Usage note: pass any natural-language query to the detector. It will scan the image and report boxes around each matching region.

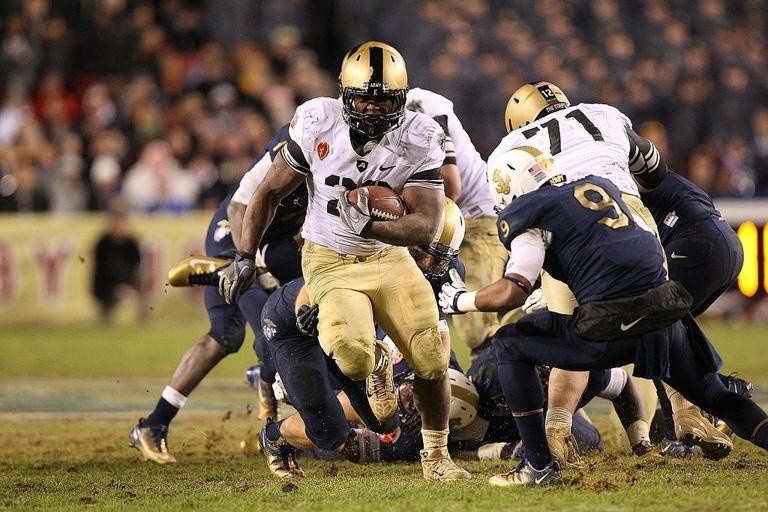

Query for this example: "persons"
[127,40,768,488]
[88,211,143,323]
[0,0,767,209]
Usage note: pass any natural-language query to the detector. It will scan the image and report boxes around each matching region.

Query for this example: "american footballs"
[349,187,404,222]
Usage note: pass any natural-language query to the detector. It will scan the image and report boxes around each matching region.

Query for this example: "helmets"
[341,41,408,139]
[395,368,478,433]
[487,146,566,216]
[408,196,465,280]
[504,81,571,133]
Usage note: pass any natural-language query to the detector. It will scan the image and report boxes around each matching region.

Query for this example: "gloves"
[296,304,319,337]
[438,268,468,315]
[522,287,547,315]
[218,251,256,305]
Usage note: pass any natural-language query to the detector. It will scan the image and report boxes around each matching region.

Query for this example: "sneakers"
[478,370,752,489]
[420,447,472,481]
[247,339,399,484]
[128,417,177,465]
[168,255,235,287]
